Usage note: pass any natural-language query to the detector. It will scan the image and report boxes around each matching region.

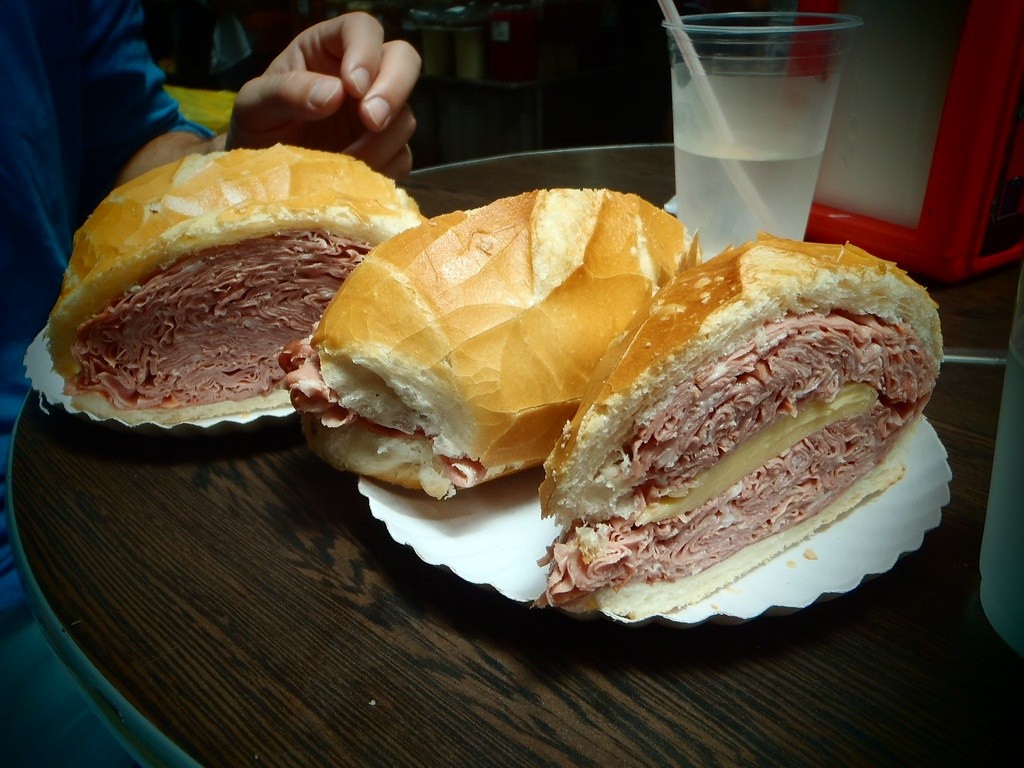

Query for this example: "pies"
[537,231,945,616]
[41,143,429,424]
[276,187,704,495]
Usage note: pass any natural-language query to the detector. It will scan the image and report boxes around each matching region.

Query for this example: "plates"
[22,320,296,430]
[358,414,953,623]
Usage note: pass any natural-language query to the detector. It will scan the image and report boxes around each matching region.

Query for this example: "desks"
[3,141,1024,768]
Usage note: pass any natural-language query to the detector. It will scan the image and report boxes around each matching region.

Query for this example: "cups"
[661,13,865,263]
[978,275,1024,655]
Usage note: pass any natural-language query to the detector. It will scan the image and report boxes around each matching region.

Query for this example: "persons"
[0,0,423,768]
